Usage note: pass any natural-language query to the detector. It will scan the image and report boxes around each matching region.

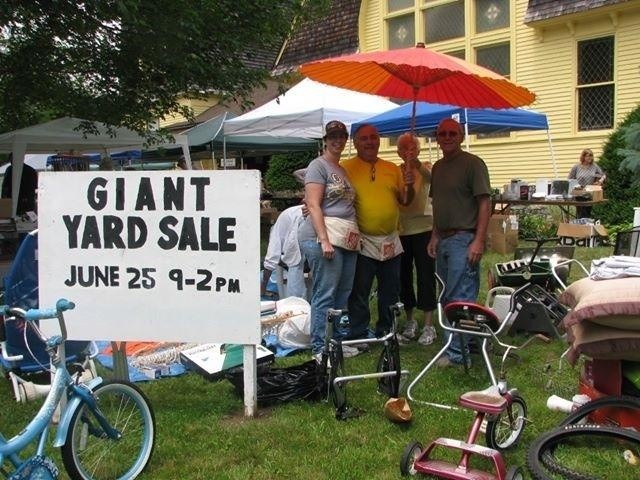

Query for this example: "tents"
[223,78,399,170]
[349,102,558,180]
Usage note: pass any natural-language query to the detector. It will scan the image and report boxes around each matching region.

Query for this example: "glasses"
[438,129,459,138]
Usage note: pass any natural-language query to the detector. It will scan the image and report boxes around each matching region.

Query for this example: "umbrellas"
[300,42,537,204]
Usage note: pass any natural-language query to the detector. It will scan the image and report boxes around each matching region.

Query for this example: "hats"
[324,121,348,136]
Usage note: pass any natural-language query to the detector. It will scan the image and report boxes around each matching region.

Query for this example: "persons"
[568,149,607,219]
[301,116,491,360]
[1,152,38,217]
[260,204,307,301]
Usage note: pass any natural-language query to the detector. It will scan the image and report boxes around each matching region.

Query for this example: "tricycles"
[399,324,551,479]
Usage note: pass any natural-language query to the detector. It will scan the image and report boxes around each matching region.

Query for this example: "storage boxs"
[487,214,518,255]
[556,221,608,248]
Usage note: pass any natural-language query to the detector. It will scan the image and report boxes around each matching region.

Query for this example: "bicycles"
[1,299,157,479]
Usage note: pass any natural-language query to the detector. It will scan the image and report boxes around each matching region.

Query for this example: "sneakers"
[311,320,472,369]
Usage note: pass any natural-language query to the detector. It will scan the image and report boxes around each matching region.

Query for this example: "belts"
[437,228,475,239]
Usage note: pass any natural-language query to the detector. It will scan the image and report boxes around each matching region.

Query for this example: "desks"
[492,196,609,222]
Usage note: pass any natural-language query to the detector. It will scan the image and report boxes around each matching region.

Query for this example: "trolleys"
[486,232,566,299]
[319,299,406,417]
